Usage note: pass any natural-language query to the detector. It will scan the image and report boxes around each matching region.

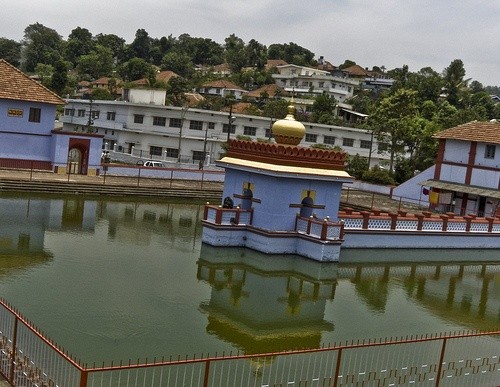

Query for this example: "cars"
[144,160,164,167]
